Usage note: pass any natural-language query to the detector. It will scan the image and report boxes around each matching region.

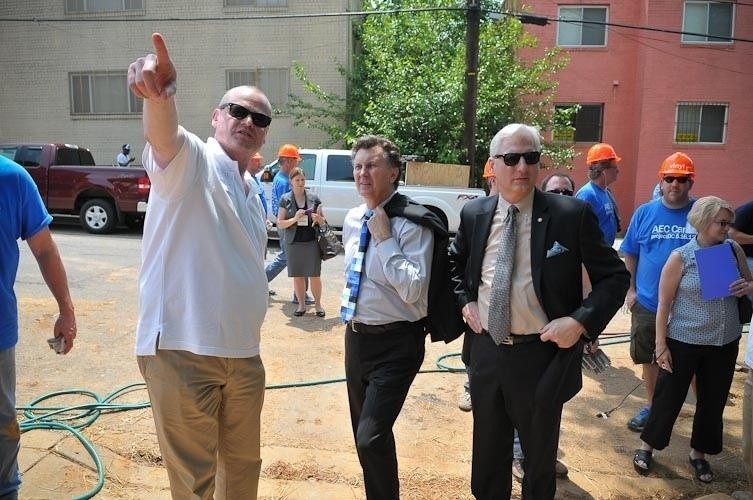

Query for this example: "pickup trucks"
[0,144,152,229]
[256,150,486,234]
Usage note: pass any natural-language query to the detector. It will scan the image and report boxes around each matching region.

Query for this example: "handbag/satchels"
[317,230,343,261]
[737,294,753,324]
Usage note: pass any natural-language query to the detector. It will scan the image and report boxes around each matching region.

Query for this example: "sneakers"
[554,459,568,479]
[511,458,525,483]
[458,388,472,411]
[292,293,315,305]
[627,406,651,431]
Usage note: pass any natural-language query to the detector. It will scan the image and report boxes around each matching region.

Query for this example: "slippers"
[632,448,652,476]
[688,454,713,482]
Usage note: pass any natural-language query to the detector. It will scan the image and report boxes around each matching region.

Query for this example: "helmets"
[250,152,263,160]
[657,152,697,176]
[586,143,622,166]
[122,143,130,150]
[482,157,496,179]
[277,144,301,161]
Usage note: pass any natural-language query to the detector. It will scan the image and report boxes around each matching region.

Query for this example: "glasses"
[217,102,271,128]
[491,147,542,166]
[546,188,574,197]
[661,175,691,183]
[714,220,732,227]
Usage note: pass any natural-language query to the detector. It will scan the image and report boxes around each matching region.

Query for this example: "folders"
[694,243,741,300]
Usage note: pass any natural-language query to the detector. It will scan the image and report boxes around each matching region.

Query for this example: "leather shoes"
[315,307,325,316]
[293,306,306,316]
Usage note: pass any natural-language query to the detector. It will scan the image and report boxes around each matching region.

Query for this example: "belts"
[345,320,410,336]
[501,334,540,346]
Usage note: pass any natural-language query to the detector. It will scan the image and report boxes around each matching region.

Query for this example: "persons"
[449,122,632,500]
[632,195,753,482]
[729,204,753,500]
[342,138,434,499]
[573,143,622,356]
[246,144,325,318]
[513,173,574,482]
[620,151,698,429]
[117,144,135,168]
[0,154,78,498]
[459,156,494,412]
[126,32,273,500]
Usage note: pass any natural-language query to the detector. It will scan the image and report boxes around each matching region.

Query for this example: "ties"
[339,208,373,325]
[487,204,520,347]
